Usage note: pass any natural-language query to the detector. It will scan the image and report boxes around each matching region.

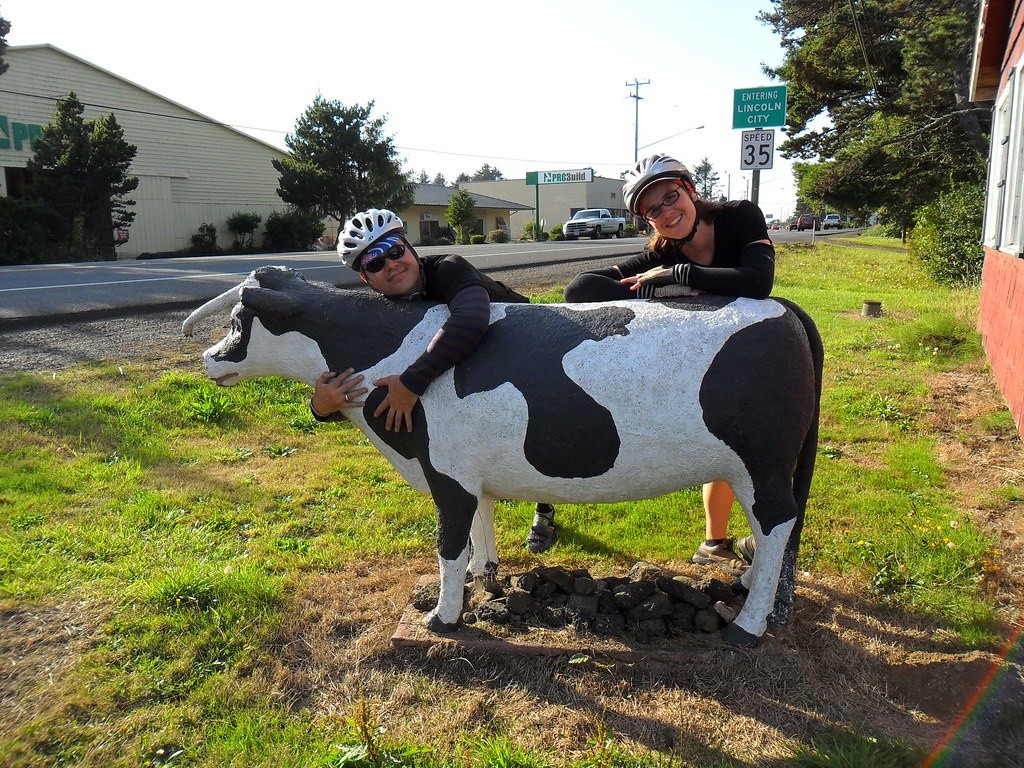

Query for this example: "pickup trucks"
[563,208,626,240]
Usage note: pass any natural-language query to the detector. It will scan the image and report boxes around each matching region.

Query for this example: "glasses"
[644,185,682,223]
[363,243,406,273]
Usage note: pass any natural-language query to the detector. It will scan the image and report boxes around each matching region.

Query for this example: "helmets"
[621,154,695,217]
[337,209,406,273]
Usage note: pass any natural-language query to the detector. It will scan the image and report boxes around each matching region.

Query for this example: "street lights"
[634,125,705,230]
[724,170,730,202]
[743,176,749,201]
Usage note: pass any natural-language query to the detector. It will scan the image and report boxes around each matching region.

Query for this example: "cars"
[771,225,779,230]
[823,214,842,229]
[785,213,821,232]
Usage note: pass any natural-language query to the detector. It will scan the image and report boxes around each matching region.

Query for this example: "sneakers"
[737,536,755,564]
[529,505,555,553]
[692,537,751,576]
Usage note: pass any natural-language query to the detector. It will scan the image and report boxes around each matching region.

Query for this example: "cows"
[180,265,824,647]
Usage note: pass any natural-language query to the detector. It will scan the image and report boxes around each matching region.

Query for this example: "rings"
[345,393,350,402]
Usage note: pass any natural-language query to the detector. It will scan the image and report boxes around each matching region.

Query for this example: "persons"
[305,208,556,553]
[564,152,777,576]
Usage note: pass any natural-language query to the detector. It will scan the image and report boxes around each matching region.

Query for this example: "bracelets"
[310,398,329,417]
